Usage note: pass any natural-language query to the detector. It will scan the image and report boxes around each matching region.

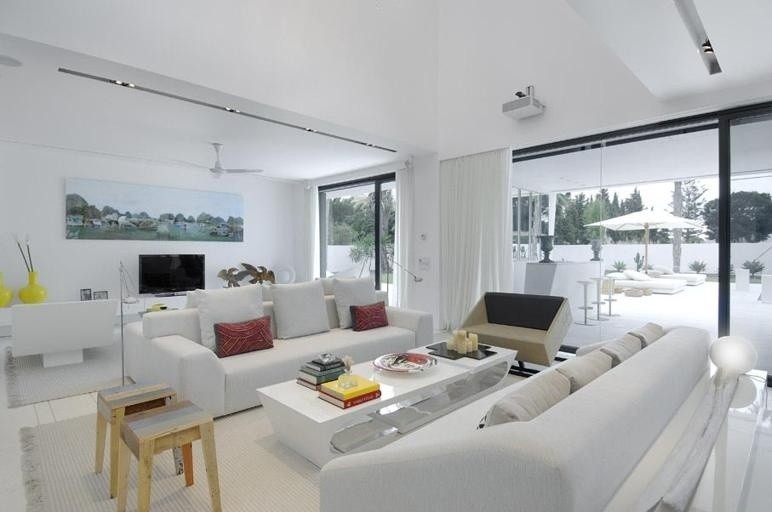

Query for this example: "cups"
[446,329,479,354]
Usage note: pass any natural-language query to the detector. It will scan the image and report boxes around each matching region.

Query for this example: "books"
[296,353,345,391]
[321,374,380,401]
[318,389,381,409]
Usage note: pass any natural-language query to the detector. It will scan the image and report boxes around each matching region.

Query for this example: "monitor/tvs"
[140,253,205,298]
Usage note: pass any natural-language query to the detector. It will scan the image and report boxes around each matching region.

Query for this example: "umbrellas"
[583,204,703,275]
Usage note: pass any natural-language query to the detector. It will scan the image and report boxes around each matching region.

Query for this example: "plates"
[373,353,438,373]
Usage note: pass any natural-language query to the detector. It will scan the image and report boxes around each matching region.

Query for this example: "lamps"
[709,334,762,397]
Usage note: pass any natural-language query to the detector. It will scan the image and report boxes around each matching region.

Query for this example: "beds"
[605,267,710,297]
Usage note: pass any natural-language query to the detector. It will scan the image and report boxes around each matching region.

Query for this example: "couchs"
[315,315,719,512]
[121,275,434,424]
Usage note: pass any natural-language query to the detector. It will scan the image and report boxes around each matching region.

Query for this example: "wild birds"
[241,263,275,284]
[217,268,252,288]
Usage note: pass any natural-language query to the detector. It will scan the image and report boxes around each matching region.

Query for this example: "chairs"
[453,287,573,378]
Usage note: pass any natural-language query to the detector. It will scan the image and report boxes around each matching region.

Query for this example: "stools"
[95,378,222,512]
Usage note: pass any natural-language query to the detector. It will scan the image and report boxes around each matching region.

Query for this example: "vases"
[0,271,49,309]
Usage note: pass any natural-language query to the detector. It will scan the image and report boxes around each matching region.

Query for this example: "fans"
[167,141,265,183]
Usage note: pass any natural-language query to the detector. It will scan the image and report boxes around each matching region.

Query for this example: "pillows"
[213,314,275,360]
[349,300,388,334]
